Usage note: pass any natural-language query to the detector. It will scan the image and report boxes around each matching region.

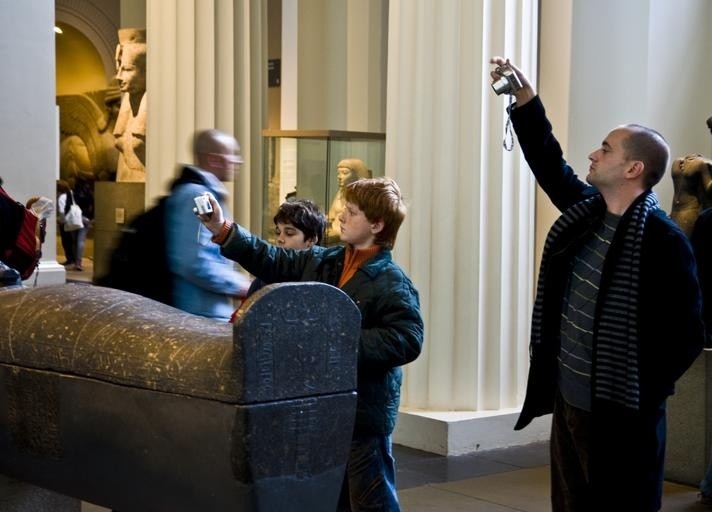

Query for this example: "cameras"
[193,196,213,217]
[491,65,523,95]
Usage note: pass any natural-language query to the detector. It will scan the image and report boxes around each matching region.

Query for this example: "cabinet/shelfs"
[261,128,386,248]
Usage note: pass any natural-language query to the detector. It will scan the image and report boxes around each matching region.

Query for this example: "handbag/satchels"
[64,204,85,232]
[0,187,53,281]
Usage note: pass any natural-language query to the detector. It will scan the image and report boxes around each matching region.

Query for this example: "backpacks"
[97,178,202,304]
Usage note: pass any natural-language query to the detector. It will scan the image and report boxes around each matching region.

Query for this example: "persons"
[487,55,706,510]
[56,180,76,266]
[113,42,146,183]
[166,128,252,320]
[677,151,711,353]
[64,174,96,271]
[321,158,368,239]
[236,195,325,317]
[184,175,425,512]
